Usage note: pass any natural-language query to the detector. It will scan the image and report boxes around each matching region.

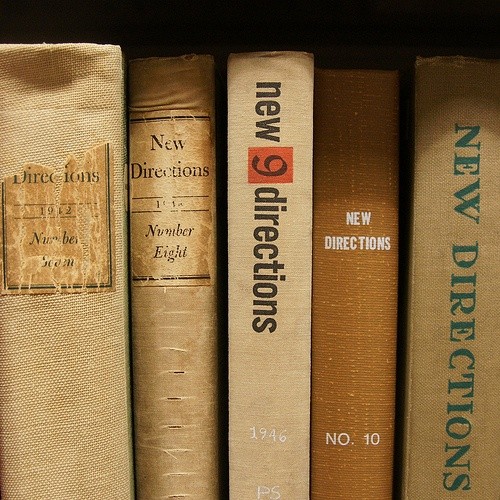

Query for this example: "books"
[0,44,500,498]
